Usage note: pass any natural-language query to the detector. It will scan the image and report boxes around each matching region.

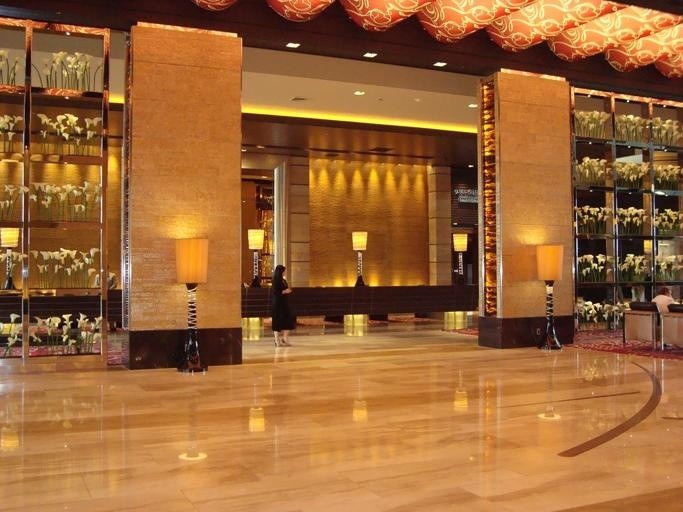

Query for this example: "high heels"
[274,338,291,347]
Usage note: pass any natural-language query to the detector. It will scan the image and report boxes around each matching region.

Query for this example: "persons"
[650,287,677,349]
[93,264,118,289]
[268,346,294,429]
[271,265,296,347]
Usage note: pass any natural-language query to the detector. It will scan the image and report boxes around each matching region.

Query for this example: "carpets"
[0,340,128,366]
[448,327,682,360]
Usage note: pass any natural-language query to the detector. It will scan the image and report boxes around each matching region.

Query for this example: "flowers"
[0,17,112,368]
[572,87,683,333]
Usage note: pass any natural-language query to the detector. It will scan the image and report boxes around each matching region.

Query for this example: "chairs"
[621,300,683,354]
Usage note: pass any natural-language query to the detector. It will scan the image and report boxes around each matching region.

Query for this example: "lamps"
[351,231,368,287]
[174,237,209,372]
[453,233,467,285]
[536,245,564,350]
[246,228,264,287]
